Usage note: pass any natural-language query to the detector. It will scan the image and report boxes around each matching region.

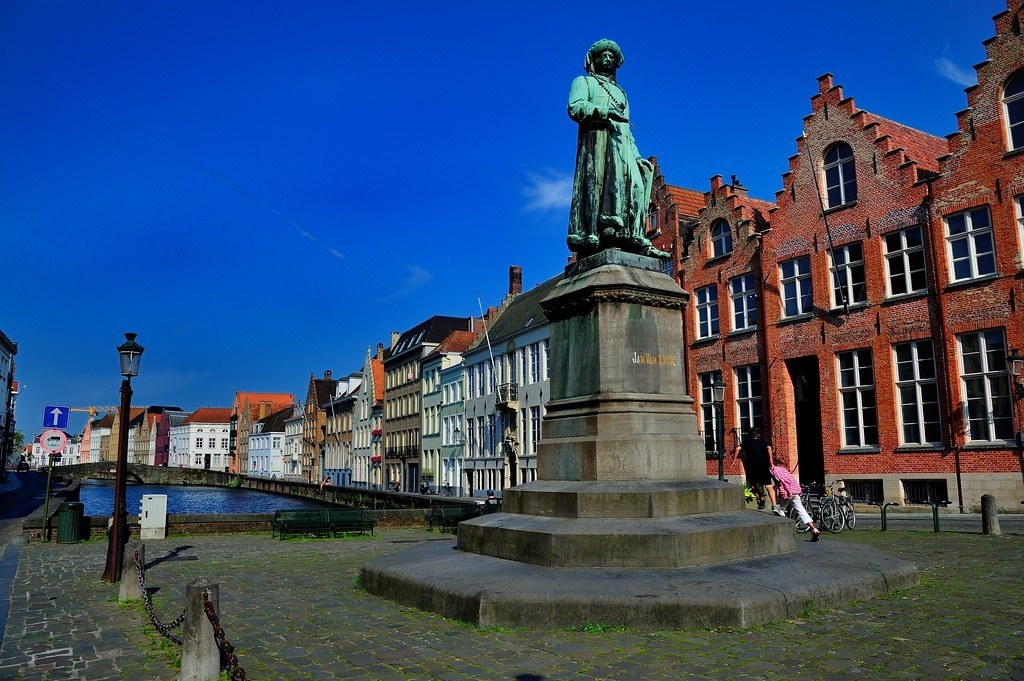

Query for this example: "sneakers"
[756,499,765,510]
[771,505,786,516]
[811,528,820,542]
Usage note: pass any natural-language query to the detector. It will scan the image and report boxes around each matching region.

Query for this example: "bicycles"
[818,479,857,534]
[789,481,822,533]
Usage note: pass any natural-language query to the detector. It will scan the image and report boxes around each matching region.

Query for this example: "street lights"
[102,332,144,579]
[710,375,727,481]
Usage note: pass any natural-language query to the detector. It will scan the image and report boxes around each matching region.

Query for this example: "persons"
[394,481,401,492]
[320,475,331,491]
[476,489,502,509]
[769,463,821,543]
[567,38,672,259]
[729,424,786,516]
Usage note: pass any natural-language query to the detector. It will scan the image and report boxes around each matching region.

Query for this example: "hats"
[486,489,494,495]
[327,477,331,479]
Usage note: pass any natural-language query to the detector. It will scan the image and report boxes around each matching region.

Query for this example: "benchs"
[424,501,502,535]
[271,508,378,541]
